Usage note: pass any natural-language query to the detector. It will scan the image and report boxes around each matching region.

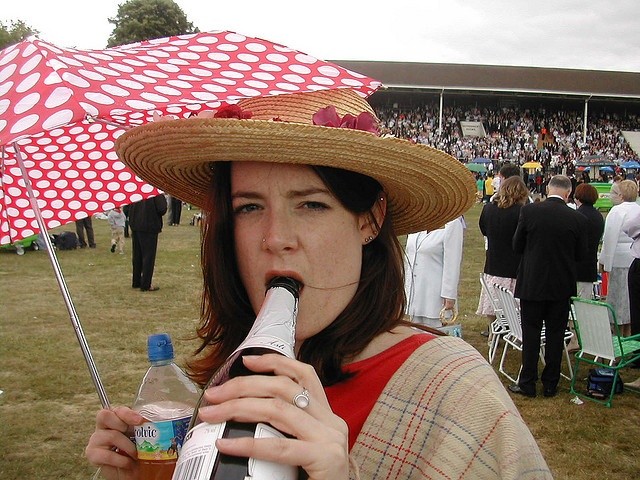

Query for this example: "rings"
[292,387,310,409]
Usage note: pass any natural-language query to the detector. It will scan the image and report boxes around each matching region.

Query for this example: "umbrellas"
[473,157,493,164]
[1,30,385,413]
[618,160,640,169]
[465,162,488,174]
[598,165,614,173]
[521,159,545,171]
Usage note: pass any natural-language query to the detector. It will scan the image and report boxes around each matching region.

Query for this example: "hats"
[115,88,476,236]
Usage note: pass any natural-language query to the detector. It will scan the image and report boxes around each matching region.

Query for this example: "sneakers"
[111,243,116,253]
[150,286,159,290]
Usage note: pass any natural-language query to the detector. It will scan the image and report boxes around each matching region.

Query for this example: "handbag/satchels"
[435,323,461,338]
[587,368,623,398]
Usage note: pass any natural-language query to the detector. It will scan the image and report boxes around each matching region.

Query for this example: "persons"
[620,211,640,392]
[119,201,131,239]
[566,183,605,354]
[600,179,640,366]
[402,215,467,334]
[170,192,182,226]
[83,89,554,479]
[166,192,173,227]
[387,105,437,147]
[506,175,589,398]
[567,104,640,160]
[122,193,168,292]
[475,175,530,347]
[476,175,485,202]
[484,172,497,203]
[107,206,126,254]
[437,102,566,158]
[76,214,97,250]
[610,175,625,207]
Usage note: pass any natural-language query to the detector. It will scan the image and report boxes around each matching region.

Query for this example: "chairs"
[493,283,575,385]
[478,275,546,365]
[568,295,640,408]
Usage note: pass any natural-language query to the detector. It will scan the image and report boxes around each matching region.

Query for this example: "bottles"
[131,333,203,480]
[171,277,299,480]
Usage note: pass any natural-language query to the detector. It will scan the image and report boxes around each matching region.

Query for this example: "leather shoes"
[508,384,536,395]
